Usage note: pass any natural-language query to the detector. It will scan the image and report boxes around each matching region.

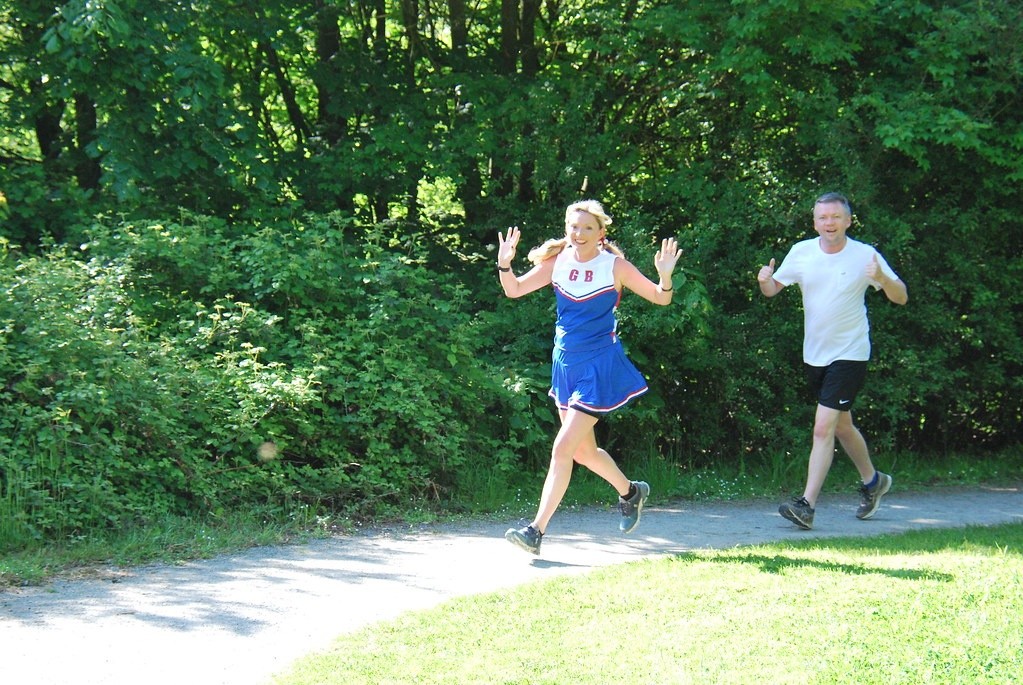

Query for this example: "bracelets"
[661,287,672,291]
[498,266,510,272]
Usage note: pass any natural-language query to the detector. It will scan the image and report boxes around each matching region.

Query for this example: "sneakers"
[612,481,650,533]
[779,496,815,530]
[855,471,891,518]
[506,525,544,555]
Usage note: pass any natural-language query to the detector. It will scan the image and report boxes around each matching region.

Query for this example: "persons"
[498,200,683,556]
[758,192,908,531]
